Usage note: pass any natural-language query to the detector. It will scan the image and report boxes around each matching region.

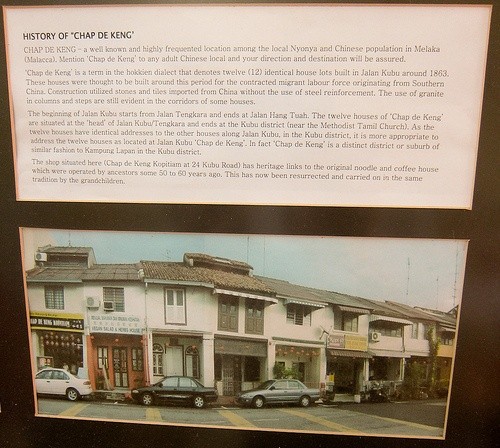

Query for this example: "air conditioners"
[103,301,115,310]
[34,252,48,262]
[369,332,381,342]
[85,295,100,309]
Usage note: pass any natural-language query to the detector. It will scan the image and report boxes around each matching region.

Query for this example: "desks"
[367,389,384,402]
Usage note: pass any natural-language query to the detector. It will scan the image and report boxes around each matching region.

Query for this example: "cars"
[131,376,220,408]
[36,366,92,401]
[236,378,321,410]
